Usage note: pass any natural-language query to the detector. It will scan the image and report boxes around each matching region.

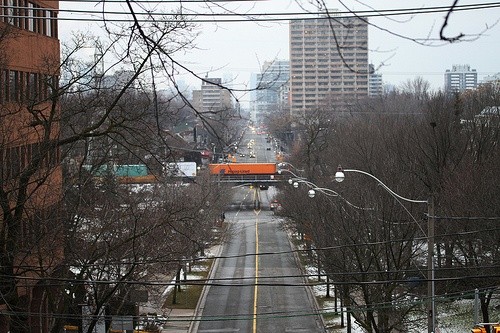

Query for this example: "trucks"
[162,162,206,179]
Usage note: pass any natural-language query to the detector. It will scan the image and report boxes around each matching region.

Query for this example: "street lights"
[308,186,384,270]
[333,164,436,333]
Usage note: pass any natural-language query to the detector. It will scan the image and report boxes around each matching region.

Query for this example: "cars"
[237,118,273,160]
[260,186,269,190]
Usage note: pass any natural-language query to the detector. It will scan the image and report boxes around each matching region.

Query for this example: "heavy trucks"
[209,162,294,181]
[80,163,158,179]
